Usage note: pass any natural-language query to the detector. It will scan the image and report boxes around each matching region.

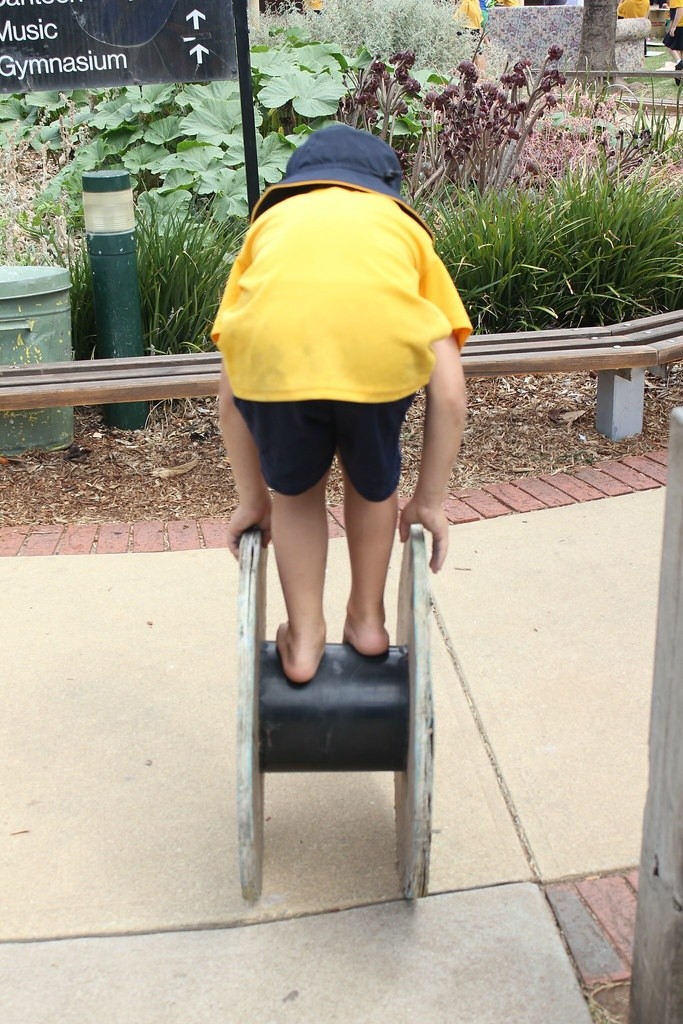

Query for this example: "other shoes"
[675,78,680,85]
[676,60,683,70]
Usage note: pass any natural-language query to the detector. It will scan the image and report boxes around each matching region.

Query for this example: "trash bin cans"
[0,266,75,458]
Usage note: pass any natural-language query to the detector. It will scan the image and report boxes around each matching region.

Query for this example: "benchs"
[514,70,683,115]
[0,309,683,441]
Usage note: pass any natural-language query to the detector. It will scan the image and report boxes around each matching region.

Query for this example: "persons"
[449,0,522,72]
[303,0,323,16]
[618,0,650,19]
[664,0,683,85]
[210,120,469,685]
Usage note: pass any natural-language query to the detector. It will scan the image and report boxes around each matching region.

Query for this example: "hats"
[251,125,434,243]
